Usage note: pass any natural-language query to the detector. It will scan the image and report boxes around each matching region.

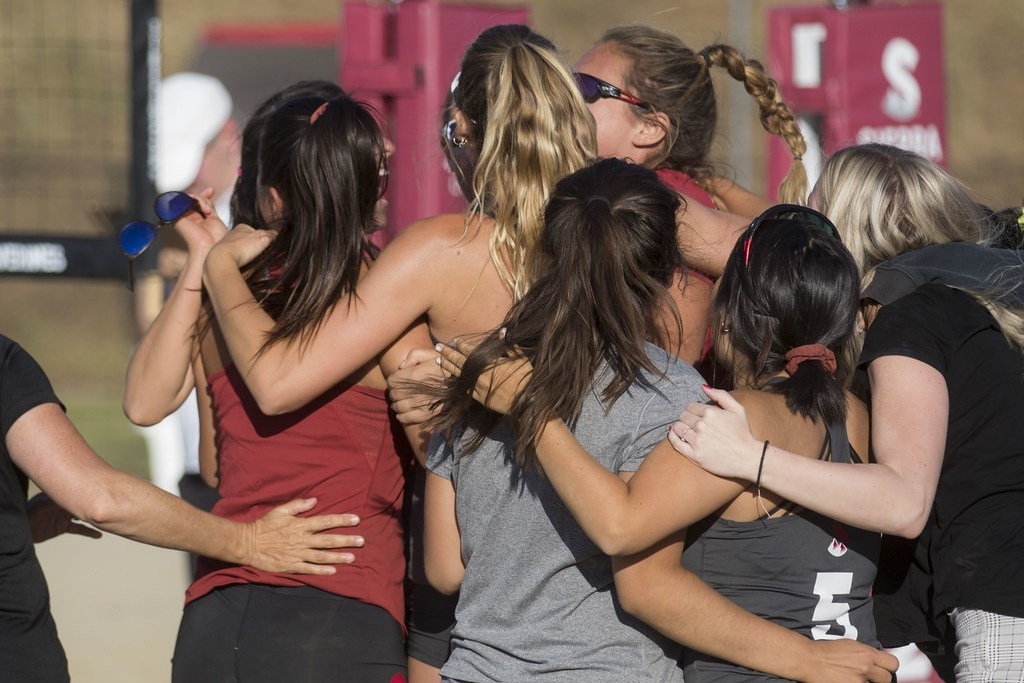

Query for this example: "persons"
[125,21,1024,683]
[0,333,365,683]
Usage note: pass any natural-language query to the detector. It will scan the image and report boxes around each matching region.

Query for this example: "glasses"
[118,191,205,290]
[744,203,843,266]
[574,73,650,110]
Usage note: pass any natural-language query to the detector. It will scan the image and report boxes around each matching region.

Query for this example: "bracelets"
[756,441,770,487]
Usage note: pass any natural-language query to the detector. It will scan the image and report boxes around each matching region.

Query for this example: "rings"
[682,429,692,442]
